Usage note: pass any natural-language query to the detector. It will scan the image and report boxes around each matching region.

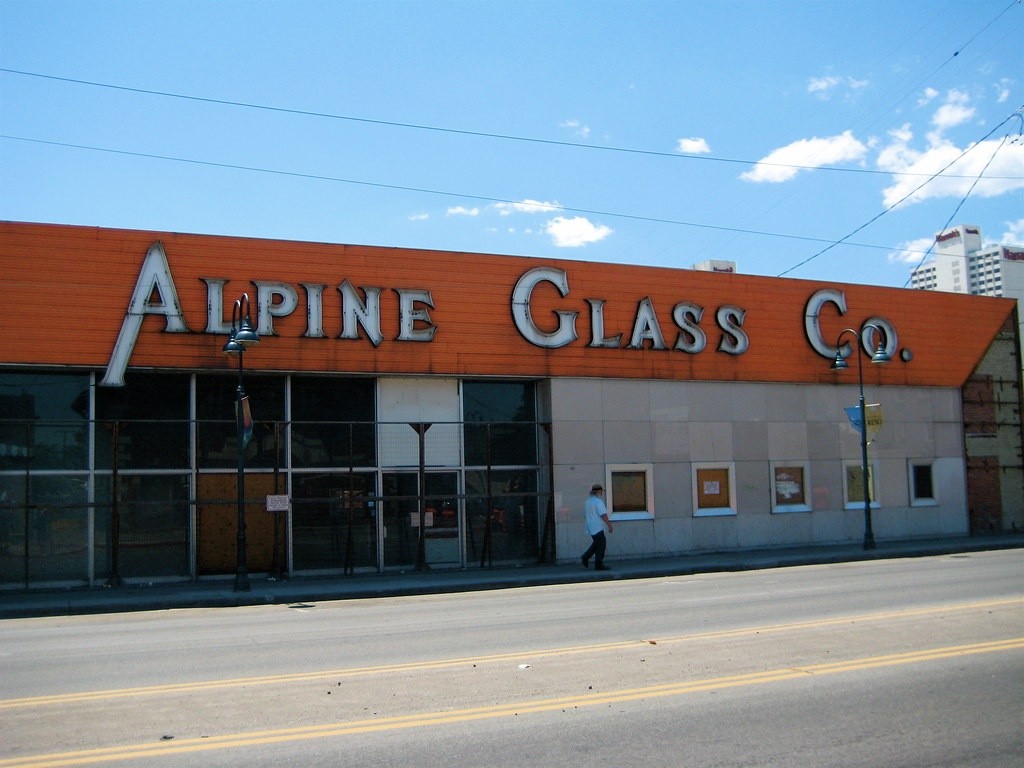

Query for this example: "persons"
[581,484,613,569]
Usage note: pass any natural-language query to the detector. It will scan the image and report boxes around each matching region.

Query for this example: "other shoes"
[595,565,610,570]
[581,555,588,568]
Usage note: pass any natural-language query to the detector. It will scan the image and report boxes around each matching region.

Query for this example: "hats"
[589,485,605,493]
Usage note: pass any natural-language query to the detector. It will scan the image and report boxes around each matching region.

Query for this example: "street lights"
[222,293,262,592]
[830,324,892,550]
